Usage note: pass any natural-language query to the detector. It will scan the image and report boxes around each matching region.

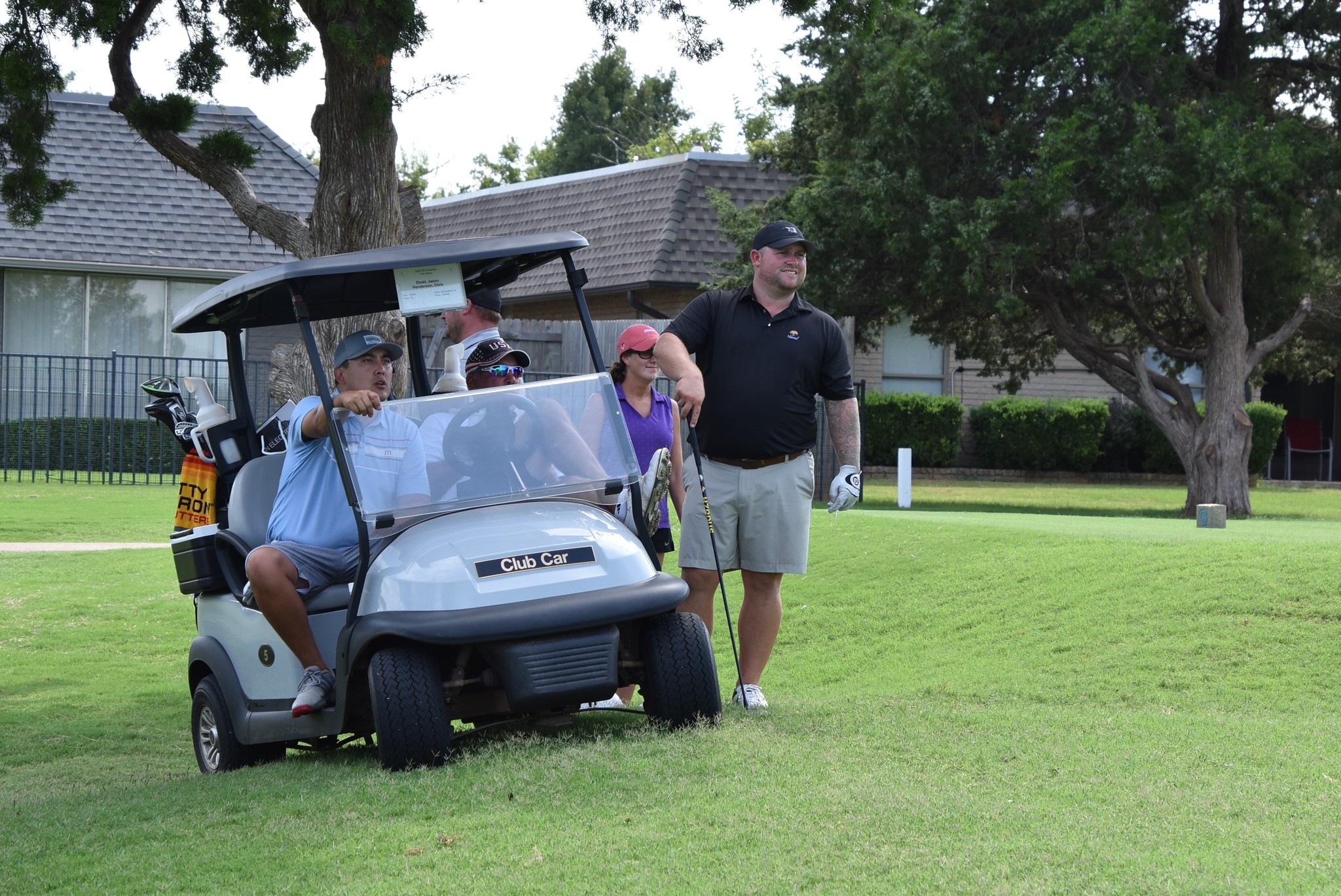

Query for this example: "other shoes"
[579,693,626,712]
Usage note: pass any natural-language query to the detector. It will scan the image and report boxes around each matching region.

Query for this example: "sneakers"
[624,447,673,536]
[732,684,768,709]
[292,665,338,718]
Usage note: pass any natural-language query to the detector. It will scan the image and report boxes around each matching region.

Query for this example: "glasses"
[627,347,654,359]
[469,364,525,378]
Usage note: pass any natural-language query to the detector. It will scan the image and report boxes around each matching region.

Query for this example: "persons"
[434,280,525,411]
[639,220,862,710]
[397,339,672,539]
[245,330,431,718]
[579,324,685,712]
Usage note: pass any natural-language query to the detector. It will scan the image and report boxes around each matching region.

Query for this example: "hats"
[753,220,816,254]
[333,330,404,369]
[464,282,501,313]
[464,339,531,376]
[617,324,660,357]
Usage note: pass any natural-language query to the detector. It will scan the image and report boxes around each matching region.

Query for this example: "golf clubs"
[173,421,221,478]
[686,430,773,717]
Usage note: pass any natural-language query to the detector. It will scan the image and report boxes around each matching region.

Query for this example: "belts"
[697,450,807,470]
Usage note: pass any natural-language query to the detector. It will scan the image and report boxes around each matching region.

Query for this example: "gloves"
[827,465,862,514]
